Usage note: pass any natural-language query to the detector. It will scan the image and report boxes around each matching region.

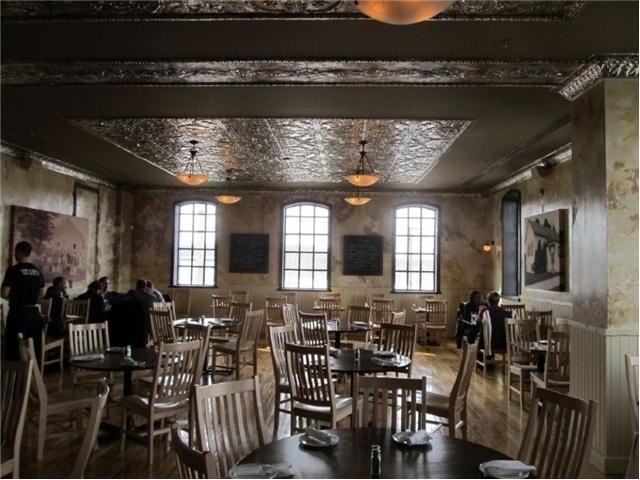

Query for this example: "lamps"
[216,169,241,204]
[483,240,494,252]
[342,140,378,187]
[176,140,207,185]
[345,187,371,205]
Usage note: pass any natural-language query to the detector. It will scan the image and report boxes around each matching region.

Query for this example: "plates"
[479,462,529,479]
[298,434,339,447]
[392,431,433,446]
[229,463,278,479]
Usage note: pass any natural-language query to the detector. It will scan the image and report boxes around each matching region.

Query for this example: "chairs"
[405,349,476,439]
[318,297,343,321]
[378,323,417,398]
[418,296,434,323]
[224,303,252,337]
[270,325,301,437]
[623,355,639,477]
[527,310,554,330]
[181,325,210,371]
[230,290,248,304]
[390,310,407,323]
[281,293,297,304]
[68,321,109,353]
[195,376,267,479]
[120,338,205,468]
[348,305,372,346]
[149,310,176,345]
[476,309,506,376]
[284,345,353,435]
[18,334,108,459]
[35,298,65,377]
[282,304,298,325]
[422,300,449,348]
[517,386,599,478]
[354,375,428,432]
[153,302,179,338]
[506,318,540,408]
[267,297,287,344]
[213,310,264,379]
[299,311,327,356]
[63,298,92,323]
[212,295,235,317]
[72,380,110,478]
[503,303,525,318]
[370,298,394,325]
[0,360,33,479]
[171,426,214,479]
[173,289,193,318]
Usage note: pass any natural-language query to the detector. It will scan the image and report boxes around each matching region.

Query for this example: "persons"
[468,293,506,371]
[456,291,489,349]
[1,241,46,373]
[124,278,158,319]
[99,277,111,304]
[146,281,165,303]
[76,281,106,324]
[41,275,69,337]
